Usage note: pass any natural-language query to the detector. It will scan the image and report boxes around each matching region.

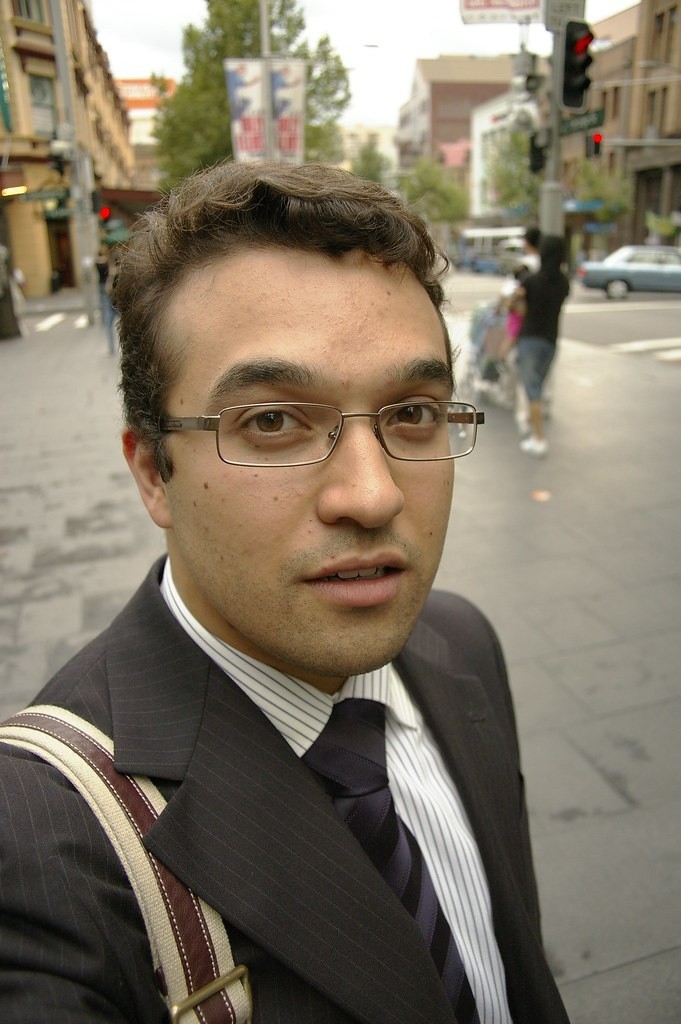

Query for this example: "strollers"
[470,291,521,410]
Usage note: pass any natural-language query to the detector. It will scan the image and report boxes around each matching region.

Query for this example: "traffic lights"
[592,130,602,154]
[98,207,112,231]
[554,17,594,115]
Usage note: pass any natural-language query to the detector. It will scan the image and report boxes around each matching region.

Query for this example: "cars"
[497,238,570,278]
[578,245,681,300]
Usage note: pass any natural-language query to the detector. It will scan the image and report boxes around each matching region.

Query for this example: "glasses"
[140,400,485,469]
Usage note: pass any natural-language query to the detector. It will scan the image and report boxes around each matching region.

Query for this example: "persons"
[88,227,571,453]
[2,162,573,1023]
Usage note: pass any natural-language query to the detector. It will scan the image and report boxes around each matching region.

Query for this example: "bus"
[450,226,532,276]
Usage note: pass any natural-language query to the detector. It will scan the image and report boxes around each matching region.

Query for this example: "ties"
[302,695,482,1023]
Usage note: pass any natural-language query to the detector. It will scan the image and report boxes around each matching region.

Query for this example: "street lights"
[258,1,380,167]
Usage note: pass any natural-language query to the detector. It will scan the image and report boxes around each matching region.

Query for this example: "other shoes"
[521,437,548,457]
